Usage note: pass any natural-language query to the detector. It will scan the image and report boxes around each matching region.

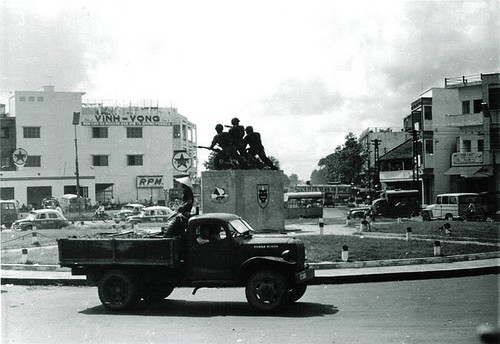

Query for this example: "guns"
[193,146,220,153]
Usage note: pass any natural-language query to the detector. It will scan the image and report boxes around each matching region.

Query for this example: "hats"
[176,177,193,187]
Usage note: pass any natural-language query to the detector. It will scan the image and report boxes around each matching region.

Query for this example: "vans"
[59,194,84,213]
[371,189,421,219]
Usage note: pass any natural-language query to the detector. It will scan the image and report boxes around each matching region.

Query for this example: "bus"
[283,191,325,219]
[286,182,353,203]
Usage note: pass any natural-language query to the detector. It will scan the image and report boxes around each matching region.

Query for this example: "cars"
[124,206,175,226]
[10,208,71,232]
[421,192,478,222]
[0,200,21,229]
[112,204,145,223]
[43,199,57,211]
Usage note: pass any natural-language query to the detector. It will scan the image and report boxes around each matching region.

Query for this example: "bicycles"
[90,211,109,223]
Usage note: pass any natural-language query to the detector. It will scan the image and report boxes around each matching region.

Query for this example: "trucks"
[56,212,315,312]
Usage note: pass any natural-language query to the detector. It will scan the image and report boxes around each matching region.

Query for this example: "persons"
[228,118,264,170]
[175,177,194,215]
[110,196,115,209]
[208,124,252,170]
[244,126,279,169]
[56,203,63,214]
[95,203,104,216]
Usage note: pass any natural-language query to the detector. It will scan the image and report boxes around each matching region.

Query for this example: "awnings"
[443,167,492,177]
